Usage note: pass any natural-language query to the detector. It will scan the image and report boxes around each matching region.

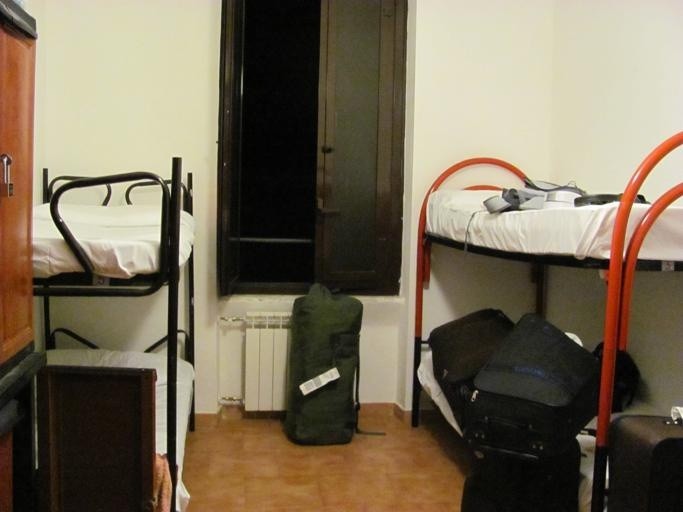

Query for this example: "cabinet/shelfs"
[0,2,38,512]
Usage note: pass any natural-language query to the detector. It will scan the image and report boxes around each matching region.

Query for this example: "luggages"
[609,413,682,512]
[427,306,600,512]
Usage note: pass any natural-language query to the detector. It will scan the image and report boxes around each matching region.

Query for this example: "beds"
[412,133,683,512]
[31,158,195,511]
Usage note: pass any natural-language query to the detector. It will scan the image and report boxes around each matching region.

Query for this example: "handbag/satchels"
[590,339,641,413]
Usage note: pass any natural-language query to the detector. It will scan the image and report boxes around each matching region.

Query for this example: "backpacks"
[283,282,363,449]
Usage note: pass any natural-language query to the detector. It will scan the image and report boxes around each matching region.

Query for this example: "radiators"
[244,313,293,413]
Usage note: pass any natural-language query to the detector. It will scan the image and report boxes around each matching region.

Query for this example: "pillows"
[433,190,503,210]
[43,348,181,385]
[32,200,163,227]
